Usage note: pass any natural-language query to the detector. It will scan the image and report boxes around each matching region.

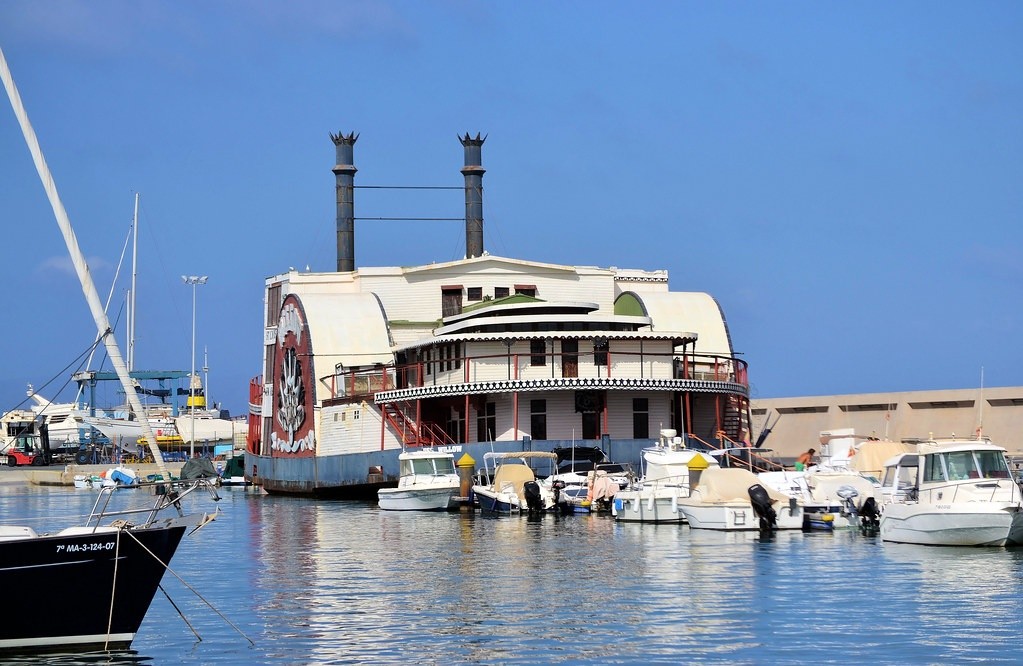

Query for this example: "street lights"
[182,275,208,458]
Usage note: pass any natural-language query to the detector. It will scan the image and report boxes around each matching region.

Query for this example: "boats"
[0,48,222,665]
[377,407,461,510]
[243,129,780,495]
[472,365,1023,547]
[1,189,249,457]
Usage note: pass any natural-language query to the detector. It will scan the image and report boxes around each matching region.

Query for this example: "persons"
[523,481,541,522]
[794,448,817,471]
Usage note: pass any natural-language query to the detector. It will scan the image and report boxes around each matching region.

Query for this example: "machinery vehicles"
[8,424,52,467]
